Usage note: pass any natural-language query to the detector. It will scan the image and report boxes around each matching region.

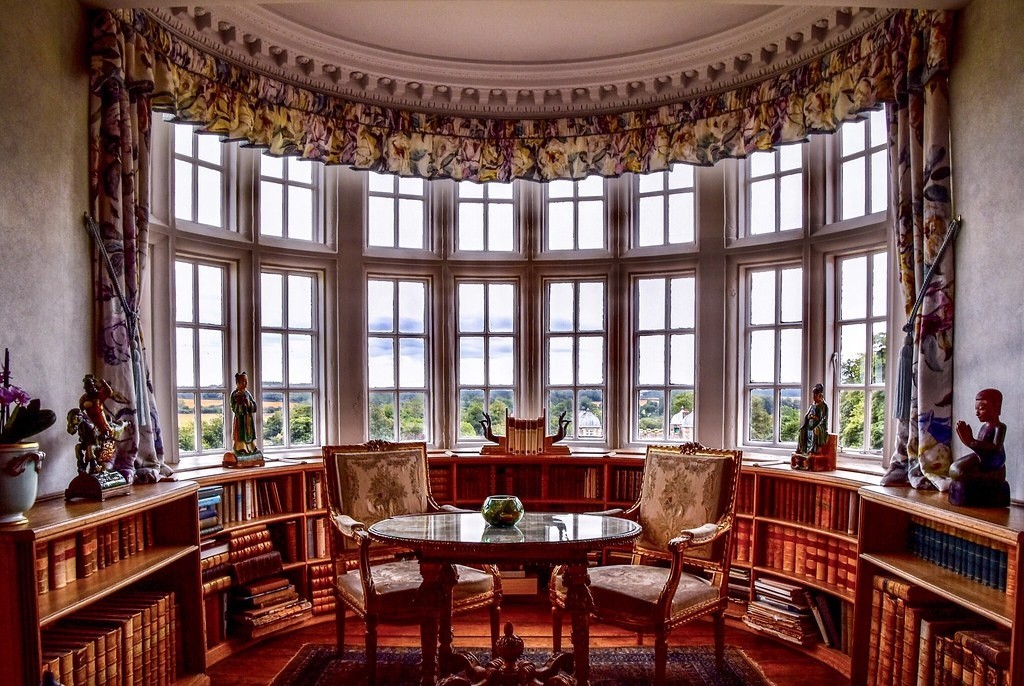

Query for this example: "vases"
[0,442,47,527]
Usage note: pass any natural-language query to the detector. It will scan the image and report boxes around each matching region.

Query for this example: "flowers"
[0,348,57,444]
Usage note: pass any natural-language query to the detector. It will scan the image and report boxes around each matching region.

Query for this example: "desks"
[367,510,643,686]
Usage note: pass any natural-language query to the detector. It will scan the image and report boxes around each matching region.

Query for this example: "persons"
[791,383,829,455]
[949,388,1007,482]
[230,371,261,454]
[78,373,113,433]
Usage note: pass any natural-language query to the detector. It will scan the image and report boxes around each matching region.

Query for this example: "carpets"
[268,641,777,686]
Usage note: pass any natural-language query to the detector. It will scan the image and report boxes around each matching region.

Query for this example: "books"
[458,463,857,656]
[200,526,312,648]
[36,511,181,686]
[305,465,450,614]
[197,476,293,535]
[865,574,1011,686]
[905,520,1007,593]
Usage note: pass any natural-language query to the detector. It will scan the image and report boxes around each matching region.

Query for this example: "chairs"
[322,439,501,686]
[547,442,744,686]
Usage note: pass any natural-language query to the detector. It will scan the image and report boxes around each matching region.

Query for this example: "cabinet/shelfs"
[167,459,325,670]
[452,447,610,601]
[315,449,457,625]
[1,481,211,686]
[851,486,1024,686]
[749,463,883,681]
[607,447,757,631]
